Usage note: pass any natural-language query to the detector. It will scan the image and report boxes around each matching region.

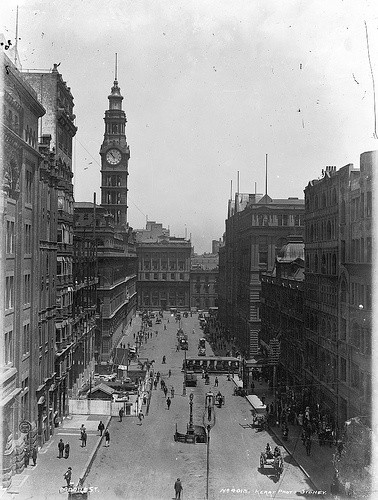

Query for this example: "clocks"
[106,149,122,165]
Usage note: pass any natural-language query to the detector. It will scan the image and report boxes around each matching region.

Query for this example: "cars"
[150,312,156,318]
[155,318,161,324]
[130,347,136,353]
[199,348,206,355]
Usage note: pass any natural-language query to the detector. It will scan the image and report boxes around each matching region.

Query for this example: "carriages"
[260,452,284,480]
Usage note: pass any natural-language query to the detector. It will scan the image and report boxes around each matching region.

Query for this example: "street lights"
[203,392,215,500]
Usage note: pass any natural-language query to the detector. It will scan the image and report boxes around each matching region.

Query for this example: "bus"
[209,307,218,314]
[181,357,241,373]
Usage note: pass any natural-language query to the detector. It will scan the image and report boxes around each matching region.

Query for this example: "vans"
[200,318,205,326]
[200,338,206,347]
[180,339,188,349]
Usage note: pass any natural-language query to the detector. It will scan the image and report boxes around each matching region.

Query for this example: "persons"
[81,431,87,447]
[151,368,170,397]
[142,394,146,404]
[138,409,144,424]
[253,392,333,456]
[80,425,86,439]
[119,307,170,355]
[216,391,224,408]
[250,369,291,392]
[161,355,166,364]
[202,313,238,357]
[119,407,124,422]
[171,386,175,398]
[166,397,171,410]
[98,421,104,436]
[214,377,219,386]
[63,467,72,487]
[31,445,37,467]
[201,368,209,384]
[58,438,64,458]
[64,441,70,459]
[328,480,338,499]
[174,477,183,500]
[104,428,110,447]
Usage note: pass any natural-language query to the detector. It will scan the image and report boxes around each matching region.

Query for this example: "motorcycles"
[282,429,287,441]
